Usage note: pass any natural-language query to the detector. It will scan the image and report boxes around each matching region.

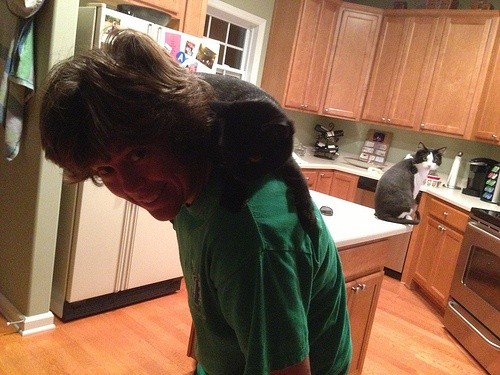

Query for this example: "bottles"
[314,124,343,153]
[445,151,463,188]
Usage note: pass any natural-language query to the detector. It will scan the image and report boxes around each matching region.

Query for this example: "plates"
[344,157,369,168]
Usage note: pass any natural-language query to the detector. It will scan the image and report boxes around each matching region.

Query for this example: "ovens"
[440,217,500,375]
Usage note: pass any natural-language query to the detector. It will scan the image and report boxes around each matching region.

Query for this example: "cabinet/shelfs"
[301,169,360,203]
[336,238,390,375]
[104,0,208,35]
[405,194,470,315]
[260,0,500,145]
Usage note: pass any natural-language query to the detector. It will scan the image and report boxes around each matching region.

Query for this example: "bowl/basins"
[117,3,172,26]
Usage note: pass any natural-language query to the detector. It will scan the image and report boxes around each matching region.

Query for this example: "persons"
[39,31,352,375]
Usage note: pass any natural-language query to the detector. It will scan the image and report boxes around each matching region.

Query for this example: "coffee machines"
[461,157,500,197]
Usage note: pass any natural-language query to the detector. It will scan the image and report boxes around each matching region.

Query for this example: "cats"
[374,141,448,224]
[192,72,320,250]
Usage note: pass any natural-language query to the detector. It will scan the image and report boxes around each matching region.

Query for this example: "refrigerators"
[50,3,220,324]
